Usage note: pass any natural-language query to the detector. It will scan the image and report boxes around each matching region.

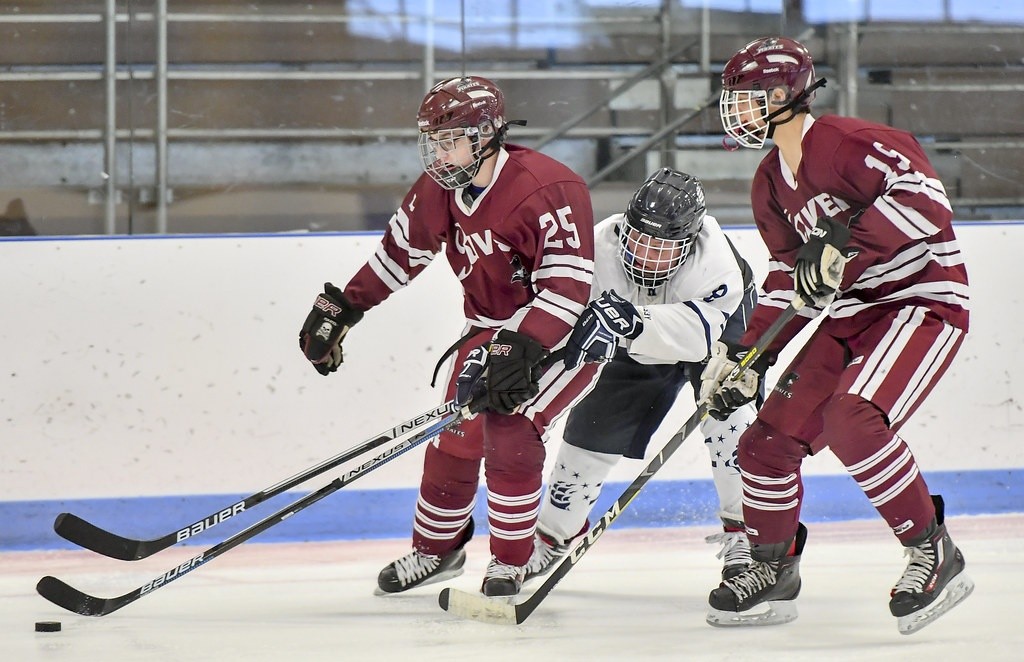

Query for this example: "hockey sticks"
[34,343,571,620]
[433,249,866,629]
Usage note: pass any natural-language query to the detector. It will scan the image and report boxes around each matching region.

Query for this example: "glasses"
[426,133,465,154]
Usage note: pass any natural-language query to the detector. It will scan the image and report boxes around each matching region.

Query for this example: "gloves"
[455,341,497,413]
[486,329,550,412]
[299,282,364,376]
[793,215,859,308]
[563,289,643,371]
[696,341,777,421]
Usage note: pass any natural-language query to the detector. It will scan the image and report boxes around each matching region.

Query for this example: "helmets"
[416,75,506,190]
[618,167,706,289]
[719,36,816,150]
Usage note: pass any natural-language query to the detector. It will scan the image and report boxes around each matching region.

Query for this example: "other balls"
[35,621,62,632]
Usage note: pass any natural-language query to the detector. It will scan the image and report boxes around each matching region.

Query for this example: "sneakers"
[373,516,474,597]
[706,522,808,628]
[479,555,526,606]
[705,511,759,582]
[889,495,974,635]
[524,520,591,586]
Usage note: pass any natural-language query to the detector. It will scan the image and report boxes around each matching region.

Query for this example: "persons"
[299,76,609,597]
[701,36,971,617]
[457,167,767,583]
